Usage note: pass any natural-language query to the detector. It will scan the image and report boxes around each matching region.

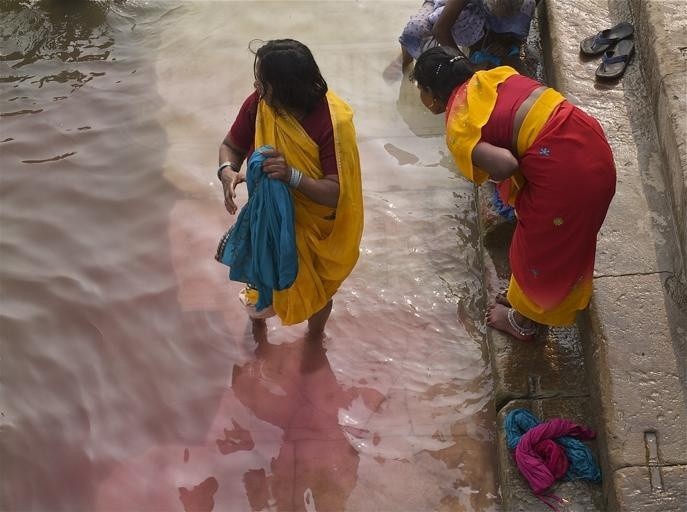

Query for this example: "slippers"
[579,21,635,57]
[595,39,634,79]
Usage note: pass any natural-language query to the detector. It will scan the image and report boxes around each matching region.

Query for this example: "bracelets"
[288,167,303,190]
[217,161,239,180]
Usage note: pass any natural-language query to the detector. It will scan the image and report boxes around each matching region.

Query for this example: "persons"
[409,45,617,342]
[209,36,367,348]
[399,0,538,80]
